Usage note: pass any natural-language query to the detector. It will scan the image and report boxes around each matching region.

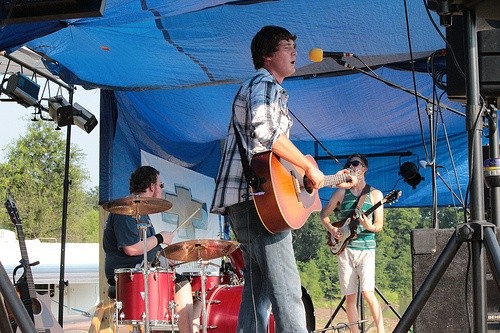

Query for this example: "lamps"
[0,56,98,134]
[398,154,425,189]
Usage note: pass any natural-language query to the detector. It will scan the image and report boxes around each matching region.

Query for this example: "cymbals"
[159,238,237,262]
[102,196,174,216]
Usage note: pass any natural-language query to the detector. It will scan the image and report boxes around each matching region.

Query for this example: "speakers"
[426,0,500,108]
[411,227,500,333]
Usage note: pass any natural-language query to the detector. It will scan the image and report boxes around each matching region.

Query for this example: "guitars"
[248,150,364,235]
[3,198,64,333]
[327,188,404,257]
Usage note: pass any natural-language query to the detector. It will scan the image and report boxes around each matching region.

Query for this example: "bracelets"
[154,234,163,246]
[332,185,336,188]
[369,225,375,231]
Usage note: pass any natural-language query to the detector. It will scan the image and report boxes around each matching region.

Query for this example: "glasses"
[154,183,164,188]
[345,161,364,168]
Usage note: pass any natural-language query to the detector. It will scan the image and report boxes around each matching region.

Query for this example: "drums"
[225,243,246,280]
[181,271,228,297]
[113,267,178,327]
[198,284,316,333]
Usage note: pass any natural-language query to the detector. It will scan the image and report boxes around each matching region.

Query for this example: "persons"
[103,166,201,333]
[319,154,385,333]
[210,26,359,333]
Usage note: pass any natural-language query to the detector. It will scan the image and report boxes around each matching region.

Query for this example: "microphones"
[419,160,444,168]
[309,48,354,62]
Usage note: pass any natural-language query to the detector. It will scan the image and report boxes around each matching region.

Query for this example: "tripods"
[322,286,402,333]
[392,6,500,333]
